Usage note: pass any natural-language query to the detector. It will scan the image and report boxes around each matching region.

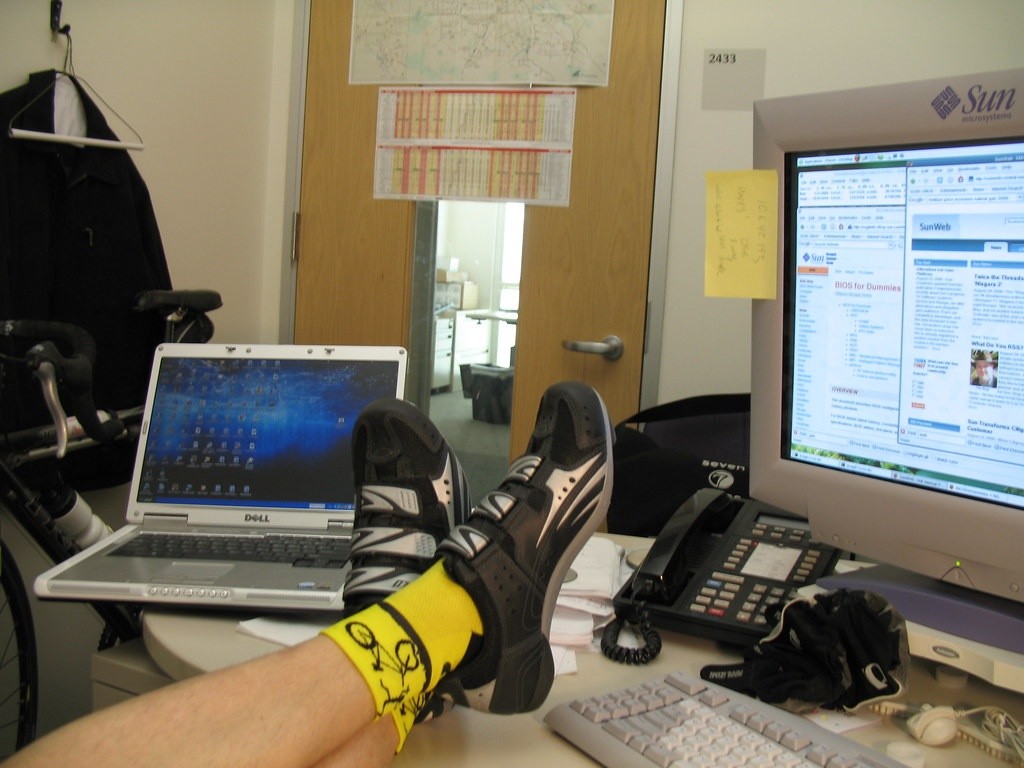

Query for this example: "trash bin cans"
[471,363,515,425]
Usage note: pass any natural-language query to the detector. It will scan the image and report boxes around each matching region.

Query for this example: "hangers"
[8,28,145,151]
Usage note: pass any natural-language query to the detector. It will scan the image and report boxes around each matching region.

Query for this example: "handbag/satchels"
[606,392,752,537]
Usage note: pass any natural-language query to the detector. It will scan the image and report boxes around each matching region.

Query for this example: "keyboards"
[543,669,912,768]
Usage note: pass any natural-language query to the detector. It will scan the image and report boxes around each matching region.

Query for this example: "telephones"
[612,487,844,648]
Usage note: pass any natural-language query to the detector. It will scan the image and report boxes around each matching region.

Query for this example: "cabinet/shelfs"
[430,318,454,393]
[443,310,491,394]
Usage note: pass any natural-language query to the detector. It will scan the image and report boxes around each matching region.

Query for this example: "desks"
[140,533,1024,768]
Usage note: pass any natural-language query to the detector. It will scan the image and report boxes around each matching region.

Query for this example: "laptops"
[34,342,408,612]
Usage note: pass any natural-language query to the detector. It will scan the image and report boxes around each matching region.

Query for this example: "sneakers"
[343,396,472,723]
[436,382,613,716]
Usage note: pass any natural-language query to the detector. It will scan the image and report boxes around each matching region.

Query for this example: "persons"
[970,351,997,387]
[0,379,617,768]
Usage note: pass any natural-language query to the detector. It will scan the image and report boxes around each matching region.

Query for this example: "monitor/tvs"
[748,68,1024,695]
